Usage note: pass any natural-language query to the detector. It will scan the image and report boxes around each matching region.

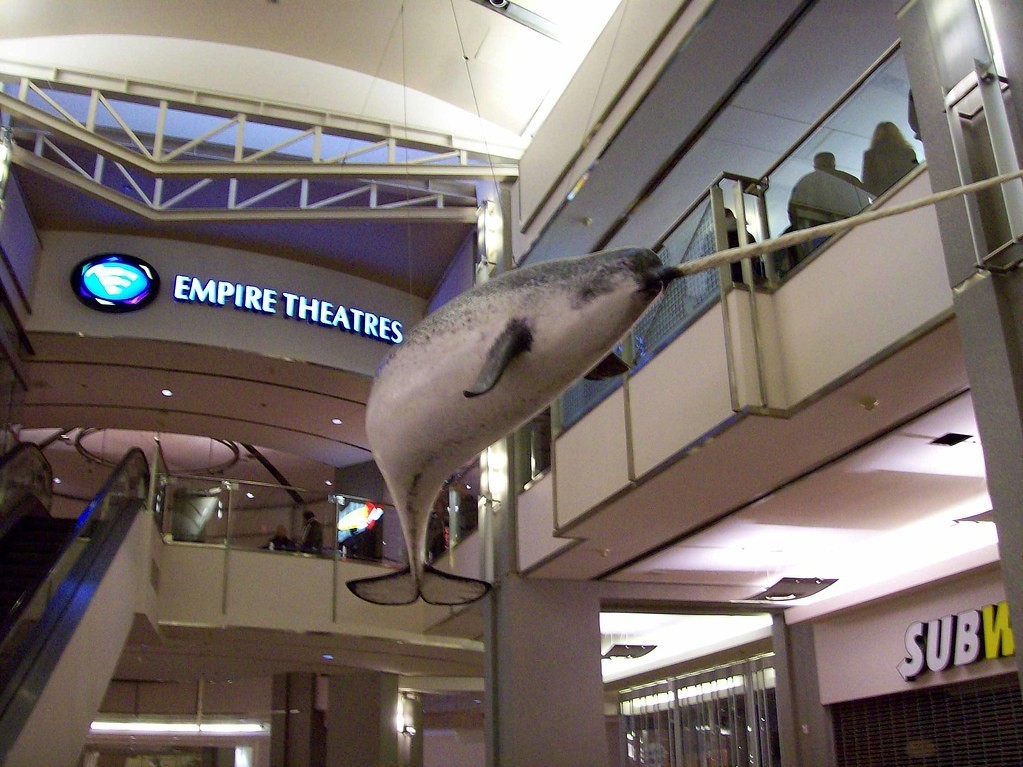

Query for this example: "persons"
[295,511,324,554]
[719,120,919,290]
[257,524,296,553]
[426,504,443,560]
[453,481,478,543]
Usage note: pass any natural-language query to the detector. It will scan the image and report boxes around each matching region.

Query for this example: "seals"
[344,246,665,606]
